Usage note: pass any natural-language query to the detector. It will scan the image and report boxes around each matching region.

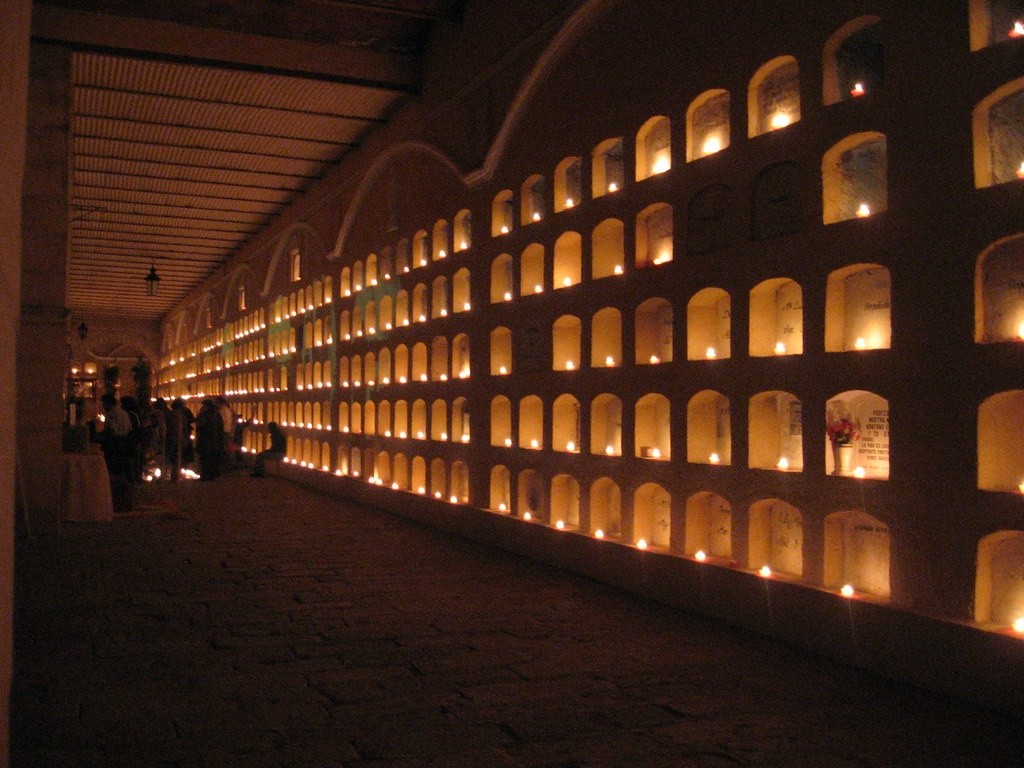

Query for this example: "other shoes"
[248,473,264,478]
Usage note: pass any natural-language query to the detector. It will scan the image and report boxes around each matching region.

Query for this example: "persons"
[99,392,253,512]
[249,421,287,478]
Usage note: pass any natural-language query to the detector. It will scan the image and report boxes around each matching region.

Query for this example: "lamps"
[144,261,162,296]
[77,323,88,341]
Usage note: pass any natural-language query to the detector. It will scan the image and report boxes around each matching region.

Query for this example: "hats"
[201,399,212,405]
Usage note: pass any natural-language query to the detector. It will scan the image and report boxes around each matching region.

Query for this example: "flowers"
[827,408,863,442]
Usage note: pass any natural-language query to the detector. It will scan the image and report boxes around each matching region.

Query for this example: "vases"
[831,443,854,474]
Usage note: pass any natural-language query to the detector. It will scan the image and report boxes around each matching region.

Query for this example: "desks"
[64,443,106,525]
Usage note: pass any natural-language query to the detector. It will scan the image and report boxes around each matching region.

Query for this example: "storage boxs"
[62,424,89,452]
